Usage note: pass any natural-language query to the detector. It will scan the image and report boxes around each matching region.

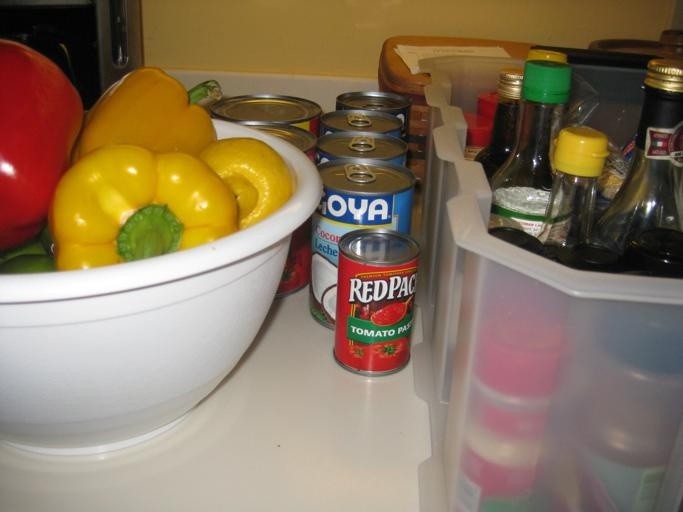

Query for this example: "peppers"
[0,34,297,273]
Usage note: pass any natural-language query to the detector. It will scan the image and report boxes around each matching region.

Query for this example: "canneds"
[335,89,412,123]
[309,156,415,329]
[210,93,321,135]
[229,122,317,299]
[317,133,410,169]
[322,109,406,136]
[334,230,422,379]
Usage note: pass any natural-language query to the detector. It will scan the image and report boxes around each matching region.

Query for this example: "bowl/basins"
[0,119,322,455]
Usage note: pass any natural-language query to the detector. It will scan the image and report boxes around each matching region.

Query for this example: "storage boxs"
[408,51,682,509]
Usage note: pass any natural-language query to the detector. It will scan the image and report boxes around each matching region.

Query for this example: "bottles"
[466,47,683,279]
